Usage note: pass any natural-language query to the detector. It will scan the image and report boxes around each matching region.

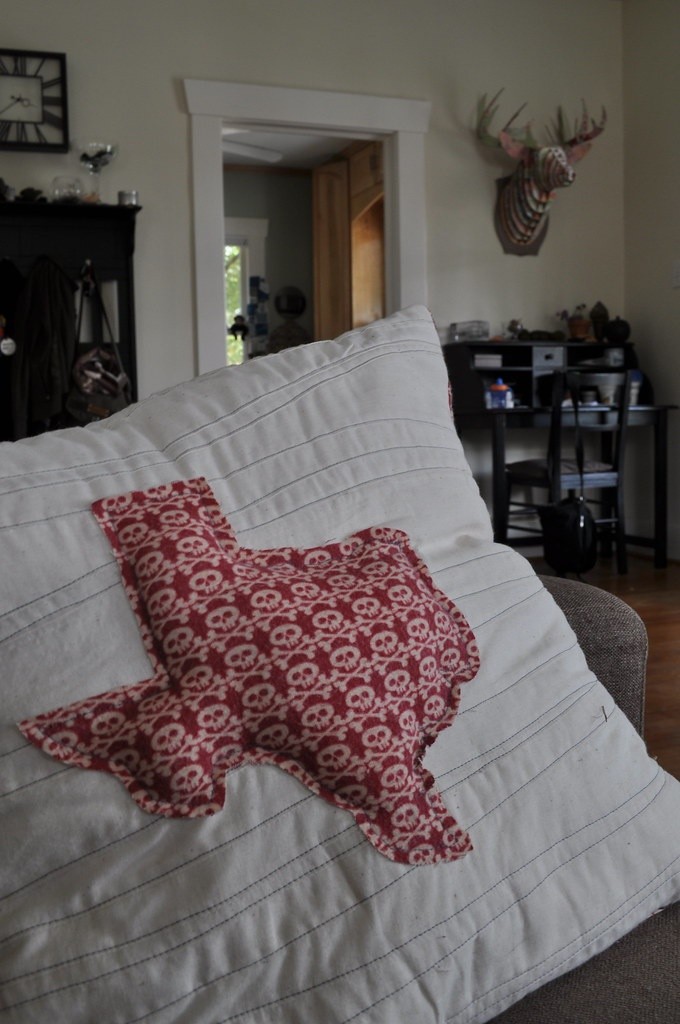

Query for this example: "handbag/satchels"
[541,498,598,575]
[65,348,132,423]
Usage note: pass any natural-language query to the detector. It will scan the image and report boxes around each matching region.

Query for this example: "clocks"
[0,44,69,153]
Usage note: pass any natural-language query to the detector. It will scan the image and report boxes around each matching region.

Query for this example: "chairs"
[509,364,636,579]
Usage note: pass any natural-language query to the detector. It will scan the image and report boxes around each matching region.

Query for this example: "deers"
[475,87,610,257]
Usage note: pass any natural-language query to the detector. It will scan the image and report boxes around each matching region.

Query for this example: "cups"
[50,175,81,202]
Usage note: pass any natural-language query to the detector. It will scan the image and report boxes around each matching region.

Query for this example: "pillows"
[0,309,680,1024]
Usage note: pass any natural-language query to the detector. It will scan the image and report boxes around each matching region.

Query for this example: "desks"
[450,403,678,571]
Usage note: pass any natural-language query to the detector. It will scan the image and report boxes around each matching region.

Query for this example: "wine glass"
[77,143,116,204]
